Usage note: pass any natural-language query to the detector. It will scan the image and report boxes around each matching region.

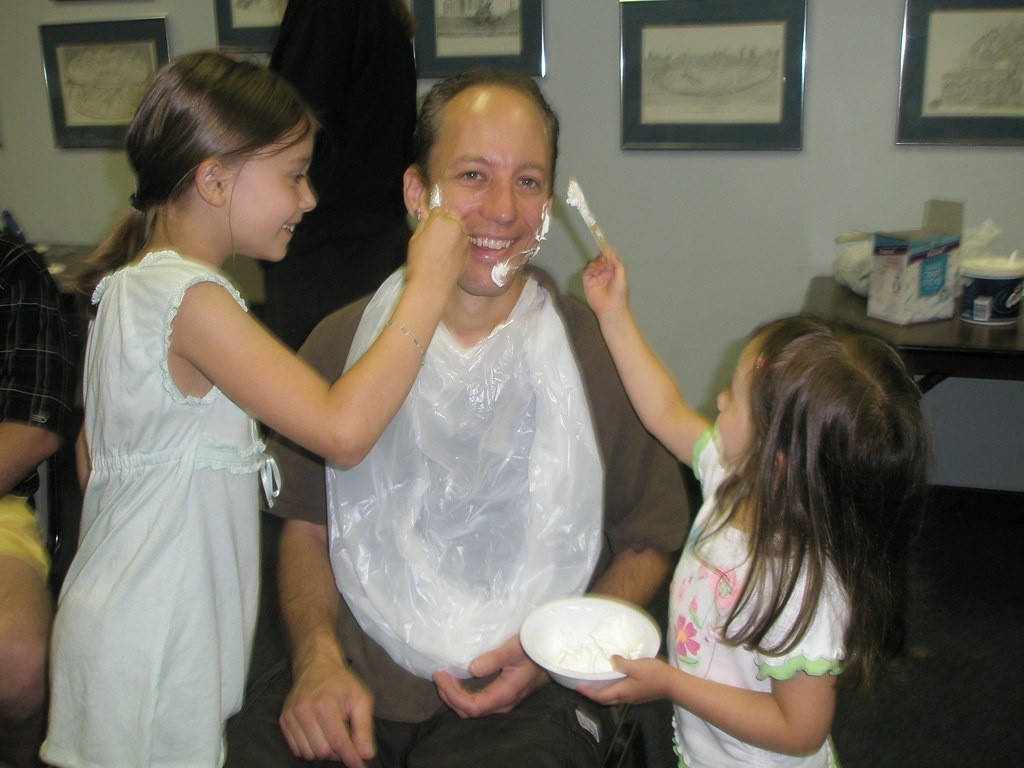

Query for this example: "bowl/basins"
[519,595,663,692]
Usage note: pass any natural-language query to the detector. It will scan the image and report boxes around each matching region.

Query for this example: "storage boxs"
[865,200,963,326]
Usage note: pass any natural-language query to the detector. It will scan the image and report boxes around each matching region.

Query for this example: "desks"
[797,275,1024,391]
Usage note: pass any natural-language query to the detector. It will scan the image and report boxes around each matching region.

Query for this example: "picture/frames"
[37,15,171,150]
[410,0,545,79]
[895,0,1024,146]
[620,0,807,150]
[215,0,290,54]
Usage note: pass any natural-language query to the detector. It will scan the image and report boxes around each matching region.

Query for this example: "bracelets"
[384,321,427,367]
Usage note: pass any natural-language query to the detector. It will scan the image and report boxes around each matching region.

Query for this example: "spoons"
[568,180,611,261]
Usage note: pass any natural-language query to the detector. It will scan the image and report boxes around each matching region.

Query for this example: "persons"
[0,212,83,728]
[230,65,688,768]
[573,245,930,768]
[36,49,463,768]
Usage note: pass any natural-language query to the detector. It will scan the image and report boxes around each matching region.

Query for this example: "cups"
[958,257,1024,327]
